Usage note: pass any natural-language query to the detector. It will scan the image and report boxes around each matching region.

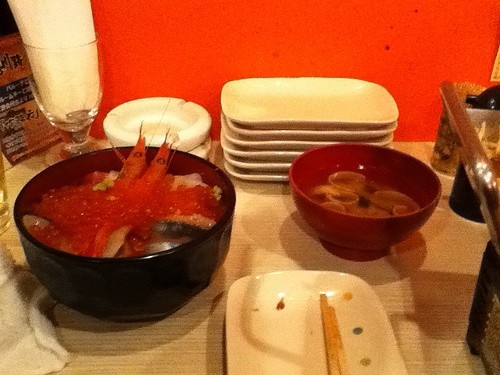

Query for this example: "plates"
[221,77,398,182]
[226,269,409,375]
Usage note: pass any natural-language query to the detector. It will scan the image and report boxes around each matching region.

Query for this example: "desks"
[0,139,492,375]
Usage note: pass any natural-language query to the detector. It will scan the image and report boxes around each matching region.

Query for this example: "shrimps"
[139,124,181,188]
[107,116,150,182]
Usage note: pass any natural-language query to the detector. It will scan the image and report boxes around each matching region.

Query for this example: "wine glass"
[19,30,105,166]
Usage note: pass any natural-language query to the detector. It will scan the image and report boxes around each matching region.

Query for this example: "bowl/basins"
[13,144,236,323]
[288,142,442,263]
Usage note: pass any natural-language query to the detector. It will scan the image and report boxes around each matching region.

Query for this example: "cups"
[428,97,461,176]
[447,159,500,223]
[0,147,13,236]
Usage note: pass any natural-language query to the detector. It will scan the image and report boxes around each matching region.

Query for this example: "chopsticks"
[320,293,350,375]
